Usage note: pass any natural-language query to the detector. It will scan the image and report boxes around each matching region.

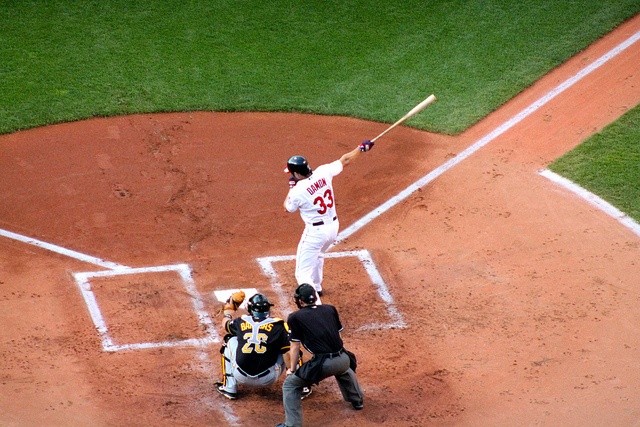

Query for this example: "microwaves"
[370,94,438,143]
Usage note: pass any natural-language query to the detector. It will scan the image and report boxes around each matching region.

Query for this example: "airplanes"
[223,291,245,310]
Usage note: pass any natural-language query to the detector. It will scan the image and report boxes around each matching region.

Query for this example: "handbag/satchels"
[223,313,232,318]
[291,369,295,372]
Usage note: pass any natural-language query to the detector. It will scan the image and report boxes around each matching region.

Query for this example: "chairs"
[217,385,236,399]
[351,398,364,409]
[300,386,312,399]
[274,422,288,426]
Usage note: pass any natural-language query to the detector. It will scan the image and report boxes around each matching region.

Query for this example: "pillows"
[248,292,274,319]
[294,282,317,308]
[287,154,309,171]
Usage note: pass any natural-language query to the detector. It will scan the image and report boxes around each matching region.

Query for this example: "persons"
[217,294,293,400]
[282,138,375,308]
[274,282,364,426]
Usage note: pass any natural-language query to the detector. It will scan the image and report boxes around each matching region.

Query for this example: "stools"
[237,367,270,378]
[313,215,337,225]
[314,348,344,358]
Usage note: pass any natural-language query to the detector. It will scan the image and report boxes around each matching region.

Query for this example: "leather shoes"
[288,176,298,188]
[359,139,374,151]
